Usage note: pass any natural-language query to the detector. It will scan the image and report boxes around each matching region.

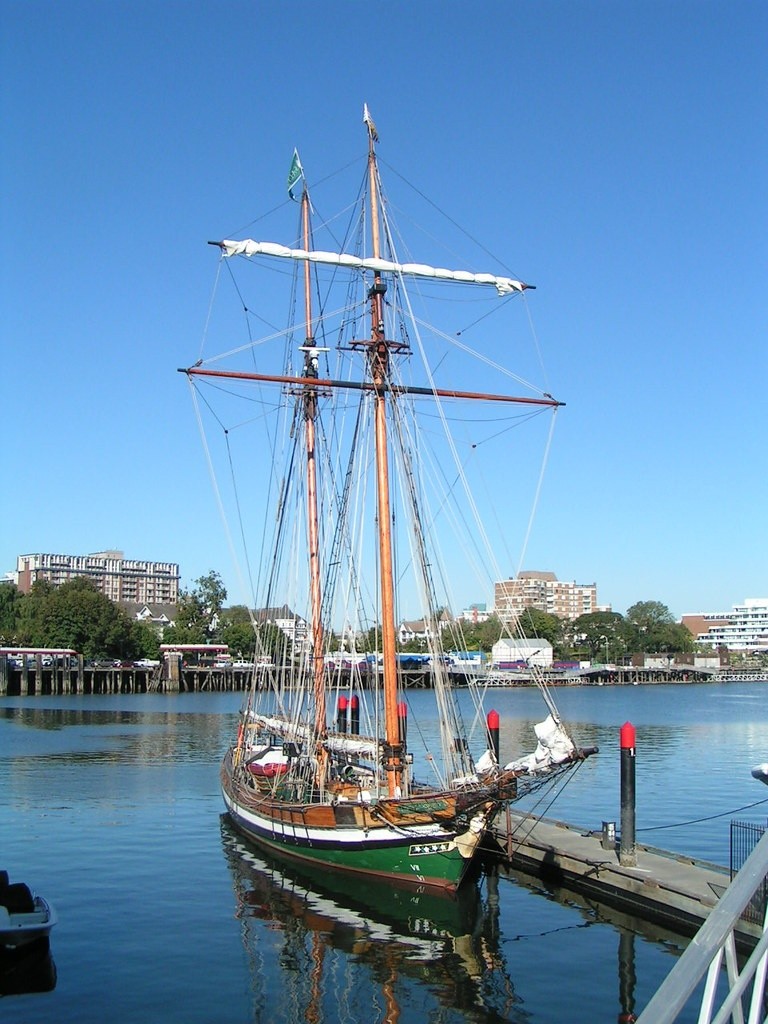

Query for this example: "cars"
[215,660,231,668]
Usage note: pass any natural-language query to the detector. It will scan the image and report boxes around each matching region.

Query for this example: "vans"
[233,660,255,668]
[91,658,115,667]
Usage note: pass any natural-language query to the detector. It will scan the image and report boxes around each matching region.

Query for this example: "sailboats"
[174,101,600,894]
[219,812,523,1024]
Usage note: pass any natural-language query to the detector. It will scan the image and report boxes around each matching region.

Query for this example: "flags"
[287,151,302,203]
[363,105,380,143]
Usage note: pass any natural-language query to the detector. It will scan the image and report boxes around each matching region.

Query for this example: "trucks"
[134,658,160,667]
